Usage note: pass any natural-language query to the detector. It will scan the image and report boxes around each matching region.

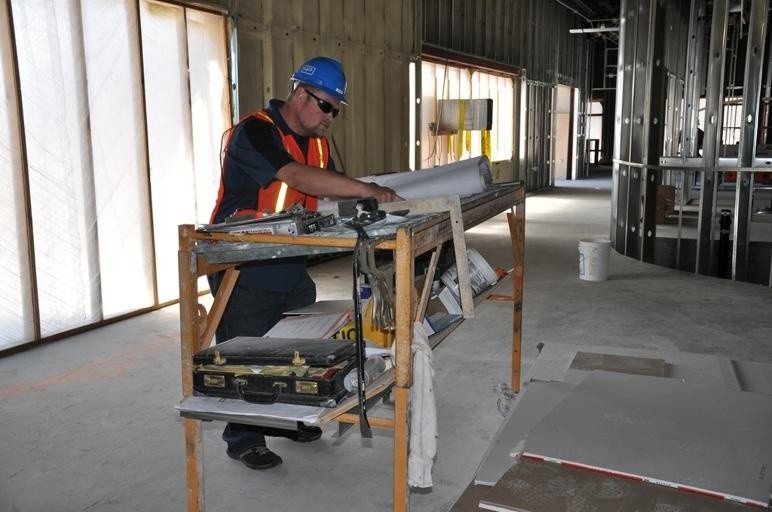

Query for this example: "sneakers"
[257,423,323,442]
[225,441,283,470]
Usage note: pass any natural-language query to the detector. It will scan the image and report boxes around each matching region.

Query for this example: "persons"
[678,119,704,185]
[205,57,406,469]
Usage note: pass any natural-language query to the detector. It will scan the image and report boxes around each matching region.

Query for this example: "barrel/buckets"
[440,248,499,303]
[577,239,612,281]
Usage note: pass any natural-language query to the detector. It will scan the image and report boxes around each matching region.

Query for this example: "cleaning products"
[360,270,373,316]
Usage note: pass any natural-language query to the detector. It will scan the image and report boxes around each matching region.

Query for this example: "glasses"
[304,88,341,118]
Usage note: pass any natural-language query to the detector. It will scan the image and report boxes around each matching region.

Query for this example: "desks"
[177,178,526,512]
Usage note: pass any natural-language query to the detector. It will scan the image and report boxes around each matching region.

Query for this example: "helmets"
[289,55,350,105]
